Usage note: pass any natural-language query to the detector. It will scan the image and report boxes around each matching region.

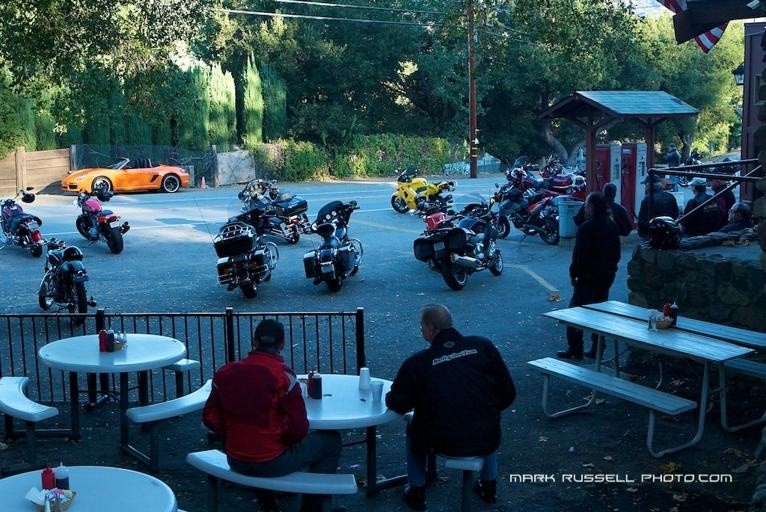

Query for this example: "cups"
[370,381,384,401]
[358,368,372,390]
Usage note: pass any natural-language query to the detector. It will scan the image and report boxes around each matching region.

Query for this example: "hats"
[254,319,285,354]
[665,143,676,149]
[687,178,706,186]
[640,174,659,184]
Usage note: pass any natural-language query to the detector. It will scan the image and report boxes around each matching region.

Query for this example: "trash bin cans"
[558,201,584,237]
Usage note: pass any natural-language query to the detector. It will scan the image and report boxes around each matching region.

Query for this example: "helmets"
[508,188,524,201]
[21,194,35,203]
[63,246,82,260]
[97,187,114,201]
[649,216,682,248]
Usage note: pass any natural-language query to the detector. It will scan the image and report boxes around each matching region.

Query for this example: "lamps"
[730,62,745,86]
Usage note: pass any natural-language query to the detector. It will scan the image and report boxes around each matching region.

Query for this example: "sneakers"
[556,350,581,358]
[583,349,603,359]
[403,483,424,507]
[473,478,497,502]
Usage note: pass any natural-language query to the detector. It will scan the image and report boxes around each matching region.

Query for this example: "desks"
[0,464,180,512]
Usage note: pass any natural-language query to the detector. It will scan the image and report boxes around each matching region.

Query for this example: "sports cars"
[60,157,189,193]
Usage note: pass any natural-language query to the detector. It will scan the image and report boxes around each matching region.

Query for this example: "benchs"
[184,449,359,512]
[0,374,59,470]
[544,299,766,443]
[446,455,484,510]
[527,356,766,456]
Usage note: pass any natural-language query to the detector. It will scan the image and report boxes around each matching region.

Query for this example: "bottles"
[646,301,678,330]
[307,370,323,399]
[41,464,55,490]
[44,494,52,511]
[55,493,62,511]
[55,461,69,489]
[99,327,127,352]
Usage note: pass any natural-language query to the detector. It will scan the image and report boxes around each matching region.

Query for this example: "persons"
[383,304,518,512]
[556,143,753,362]
[200,317,343,511]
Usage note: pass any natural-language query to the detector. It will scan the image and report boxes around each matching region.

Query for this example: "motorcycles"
[211,209,278,297]
[72,189,129,254]
[390,155,586,299]
[37,238,97,325]
[0,187,45,257]
[676,152,737,189]
[300,200,363,292]
[237,176,309,245]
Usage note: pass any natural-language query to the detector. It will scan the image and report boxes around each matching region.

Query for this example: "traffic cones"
[199,176,206,188]
